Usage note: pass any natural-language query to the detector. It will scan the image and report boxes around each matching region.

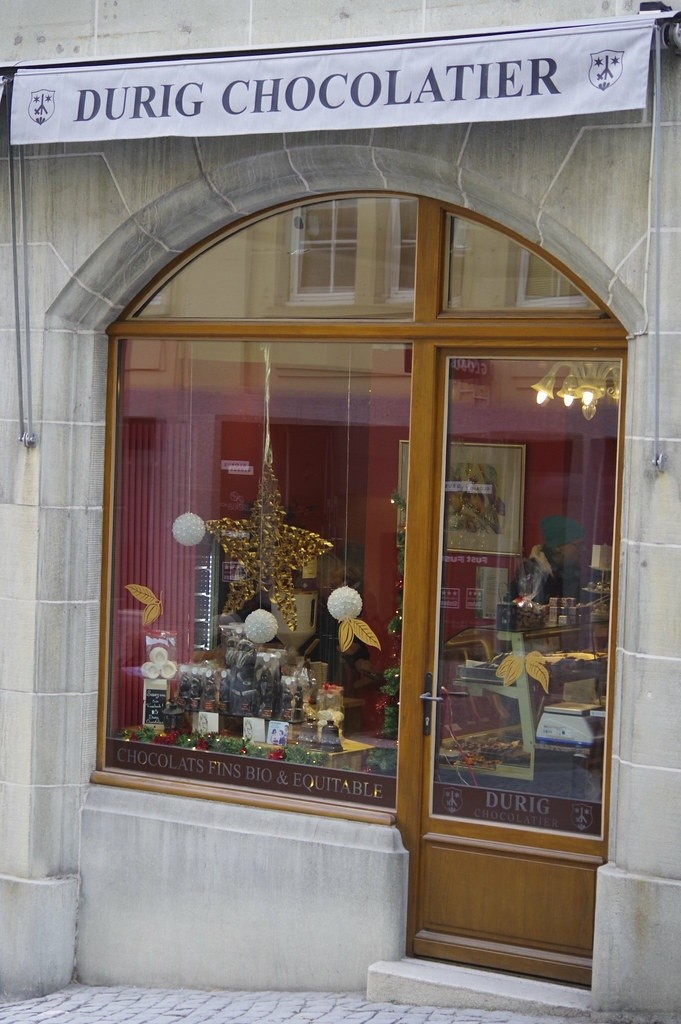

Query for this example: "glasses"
[570,538,586,551]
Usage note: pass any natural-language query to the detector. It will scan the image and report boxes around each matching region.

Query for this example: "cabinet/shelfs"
[437,622,607,782]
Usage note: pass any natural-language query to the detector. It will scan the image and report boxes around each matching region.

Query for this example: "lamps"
[591,545,613,605]
[531,361,620,420]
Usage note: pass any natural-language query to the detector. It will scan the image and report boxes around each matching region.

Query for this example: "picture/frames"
[397,440,525,559]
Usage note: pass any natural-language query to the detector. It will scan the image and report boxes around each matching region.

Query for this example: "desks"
[123,725,375,770]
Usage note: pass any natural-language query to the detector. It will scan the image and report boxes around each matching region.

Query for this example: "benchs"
[305,698,369,733]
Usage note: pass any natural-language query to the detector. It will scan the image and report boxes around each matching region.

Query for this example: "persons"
[497,516,585,666]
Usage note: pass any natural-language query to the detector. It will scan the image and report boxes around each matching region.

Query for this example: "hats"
[539,515,585,549]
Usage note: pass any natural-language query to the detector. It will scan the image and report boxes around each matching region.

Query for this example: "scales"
[537,701,605,747]
